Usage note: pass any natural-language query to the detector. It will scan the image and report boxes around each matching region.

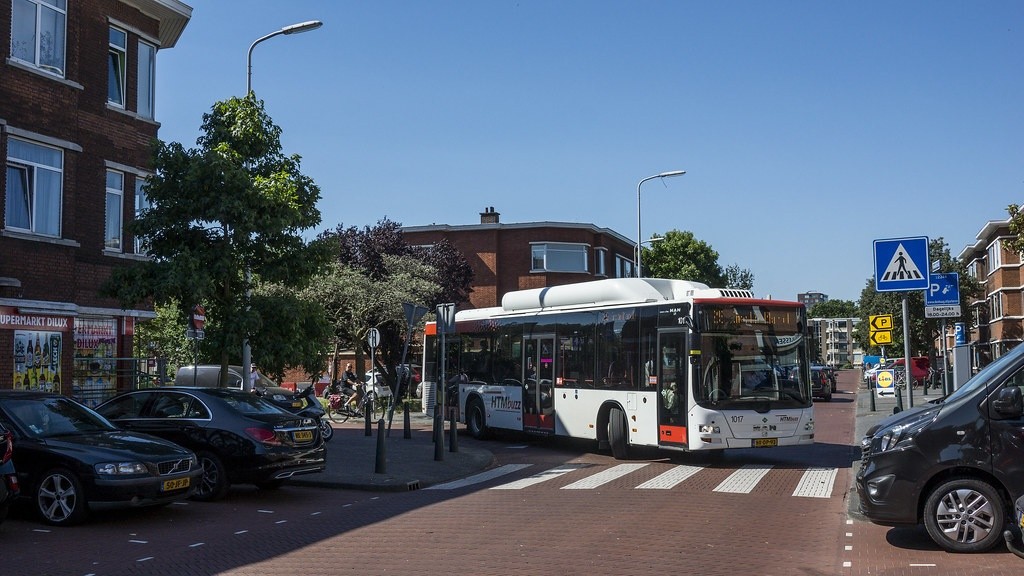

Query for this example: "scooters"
[262,378,334,443]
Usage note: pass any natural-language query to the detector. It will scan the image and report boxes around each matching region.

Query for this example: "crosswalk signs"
[873,236,931,291]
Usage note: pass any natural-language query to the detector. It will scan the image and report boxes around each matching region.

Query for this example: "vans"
[174,365,304,409]
[862,358,904,384]
[870,356,931,384]
[856,341,1024,554]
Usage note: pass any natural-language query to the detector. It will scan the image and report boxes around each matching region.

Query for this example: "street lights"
[637,171,686,277]
[633,238,665,276]
[240,20,323,391]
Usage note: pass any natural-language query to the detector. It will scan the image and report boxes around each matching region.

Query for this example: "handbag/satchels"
[329,396,341,409]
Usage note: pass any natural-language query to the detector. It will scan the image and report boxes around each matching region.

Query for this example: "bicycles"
[328,381,385,424]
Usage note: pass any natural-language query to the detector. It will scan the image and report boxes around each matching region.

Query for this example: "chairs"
[162,398,185,417]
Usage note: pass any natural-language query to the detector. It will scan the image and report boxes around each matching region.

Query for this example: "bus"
[422,277,815,459]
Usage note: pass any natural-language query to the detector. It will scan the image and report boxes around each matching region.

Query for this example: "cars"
[323,365,422,402]
[821,366,839,393]
[788,366,831,402]
[0,390,204,526]
[92,386,327,502]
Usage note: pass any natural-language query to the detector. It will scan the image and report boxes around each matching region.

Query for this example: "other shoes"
[343,404,349,412]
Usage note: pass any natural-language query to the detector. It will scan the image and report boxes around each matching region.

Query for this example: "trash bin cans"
[296,381,314,394]
[280,382,295,394]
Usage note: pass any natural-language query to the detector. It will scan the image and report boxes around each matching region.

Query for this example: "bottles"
[42,333,50,367]
[26,333,33,367]
[34,333,42,368]
[15,363,60,393]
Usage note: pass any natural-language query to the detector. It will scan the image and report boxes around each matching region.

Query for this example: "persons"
[340,362,365,416]
[241,363,259,388]
[479,340,491,354]
